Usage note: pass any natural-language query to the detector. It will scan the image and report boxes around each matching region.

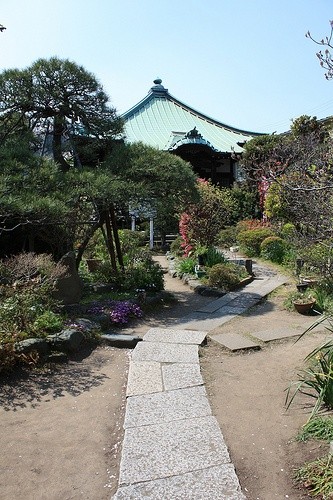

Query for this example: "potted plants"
[292,291,317,314]
